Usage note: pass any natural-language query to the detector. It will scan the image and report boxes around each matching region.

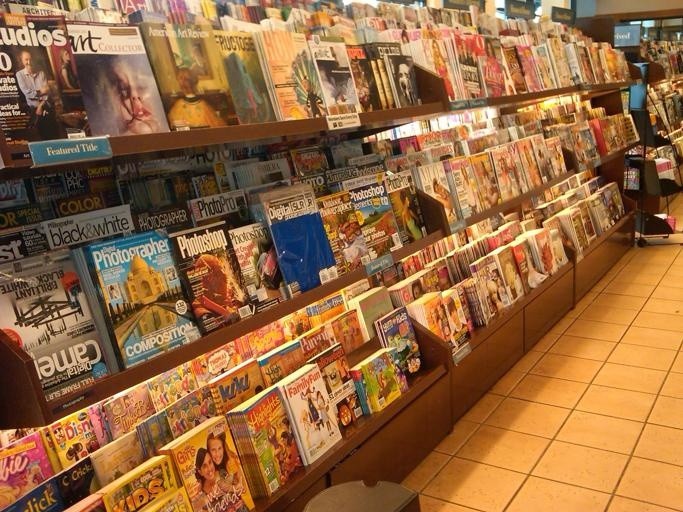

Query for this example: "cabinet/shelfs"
[0,0,683,512]
